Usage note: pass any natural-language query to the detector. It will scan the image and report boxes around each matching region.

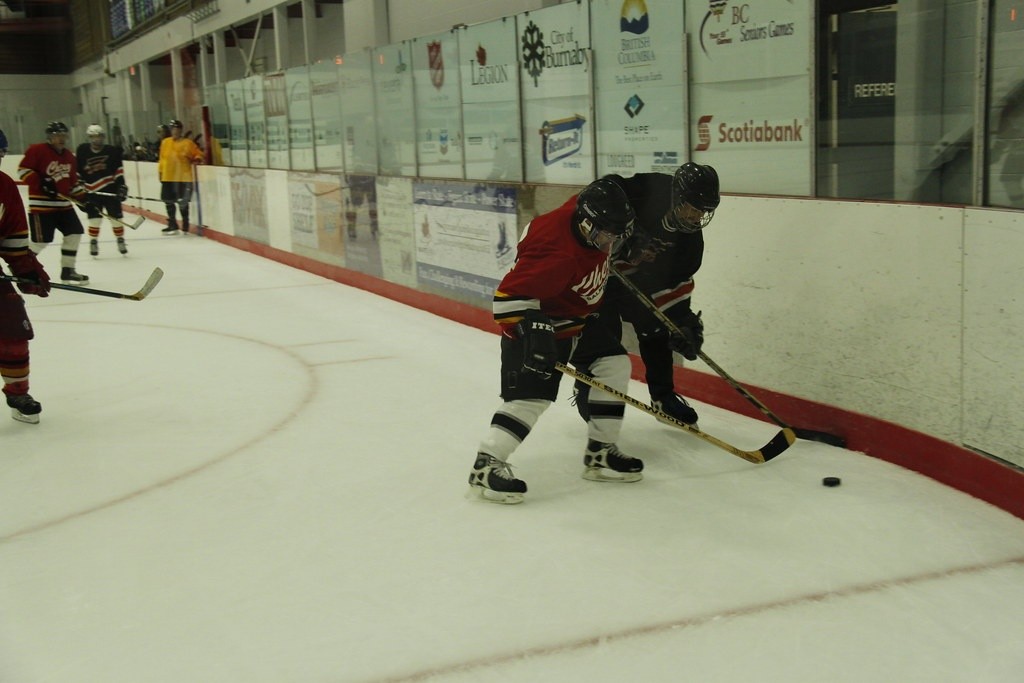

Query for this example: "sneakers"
[581,438,644,483]
[5,394,42,424]
[117,237,128,258]
[161,219,179,235]
[650,390,699,432]
[60,267,89,286]
[568,385,589,424]
[465,452,527,505]
[89,239,99,259]
[182,218,189,235]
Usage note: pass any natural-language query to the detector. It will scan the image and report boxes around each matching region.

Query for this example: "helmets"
[45,121,69,133]
[0,130,9,150]
[169,120,183,128]
[571,178,634,253]
[86,125,108,137]
[668,162,721,234]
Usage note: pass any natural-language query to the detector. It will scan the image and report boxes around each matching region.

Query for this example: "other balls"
[824,477,840,486]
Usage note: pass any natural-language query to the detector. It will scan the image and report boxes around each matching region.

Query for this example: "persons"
[0,128,50,423]
[345,175,380,244]
[599,162,720,430]
[75,125,130,260]
[132,124,170,161]
[158,121,205,235]
[466,178,644,504]
[17,122,90,285]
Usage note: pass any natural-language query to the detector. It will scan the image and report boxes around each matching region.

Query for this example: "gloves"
[116,185,128,202]
[671,310,704,361]
[8,249,51,297]
[515,308,557,382]
[41,175,58,198]
[84,192,104,219]
[630,313,671,349]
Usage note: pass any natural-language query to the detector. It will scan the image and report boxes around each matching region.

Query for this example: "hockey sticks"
[193,164,202,236]
[84,186,191,204]
[609,262,849,448]
[193,133,202,143]
[1,267,165,302]
[184,130,192,138]
[43,186,146,230]
[414,190,476,207]
[553,362,797,466]
[304,178,374,197]
